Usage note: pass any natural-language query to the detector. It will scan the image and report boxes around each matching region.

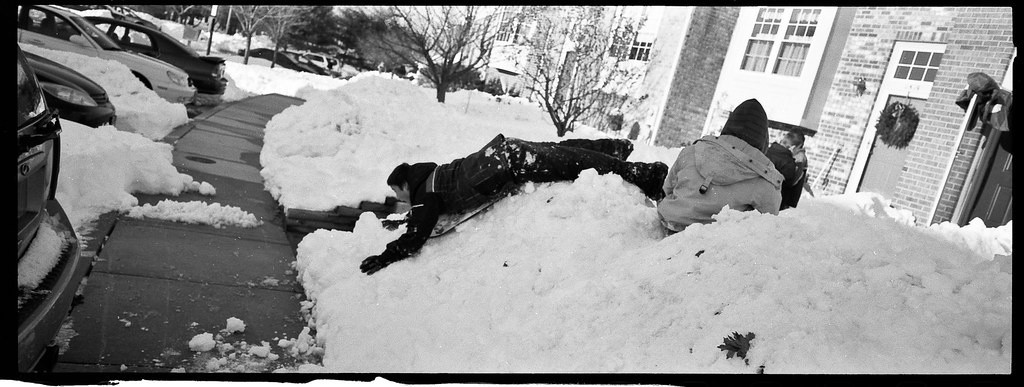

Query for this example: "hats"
[721,99,769,151]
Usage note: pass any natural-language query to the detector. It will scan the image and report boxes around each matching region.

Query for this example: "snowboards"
[429,188,517,238]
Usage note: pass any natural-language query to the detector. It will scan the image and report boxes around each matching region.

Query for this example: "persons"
[789,138,808,208]
[657,99,786,234]
[359,133,669,275]
[765,128,805,211]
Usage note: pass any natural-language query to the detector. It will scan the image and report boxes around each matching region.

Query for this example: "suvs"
[16,43,84,372]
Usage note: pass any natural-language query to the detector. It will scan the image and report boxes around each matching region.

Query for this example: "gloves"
[360,248,402,275]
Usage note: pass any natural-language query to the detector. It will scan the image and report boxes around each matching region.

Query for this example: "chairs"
[39,17,56,35]
[112,33,118,41]
[121,35,130,44]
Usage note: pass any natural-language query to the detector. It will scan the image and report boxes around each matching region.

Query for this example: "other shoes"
[614,138,634,160]
[645,162,666,201]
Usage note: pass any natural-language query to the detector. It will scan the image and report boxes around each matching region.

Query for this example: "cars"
[237,47,344,79]
[17,4,199,107]
[20,50,118,129]
[55,15,229,95]
